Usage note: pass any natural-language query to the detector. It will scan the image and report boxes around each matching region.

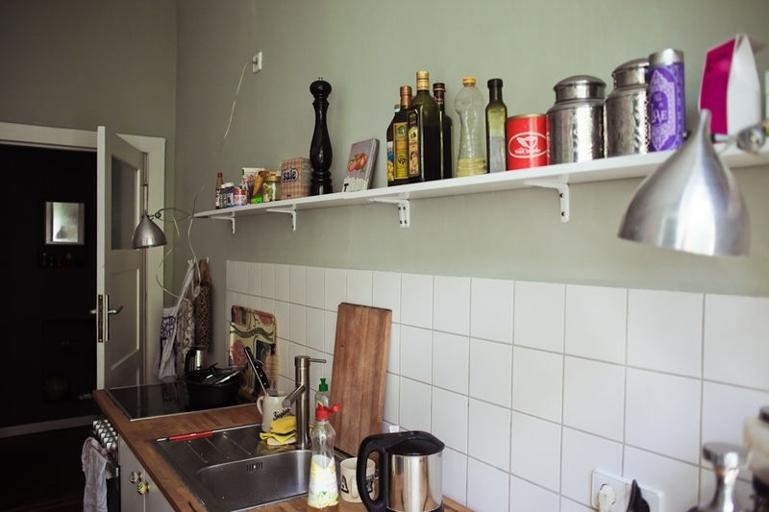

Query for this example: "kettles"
[356,430,444,511]
[183,345,208,377]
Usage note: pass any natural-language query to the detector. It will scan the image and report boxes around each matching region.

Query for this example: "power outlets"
[592,469,626,512]
[251,51,264,73]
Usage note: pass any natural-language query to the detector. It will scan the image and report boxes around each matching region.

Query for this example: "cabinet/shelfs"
[116,433,176,512]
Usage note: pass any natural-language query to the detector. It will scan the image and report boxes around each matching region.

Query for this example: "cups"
[262,176,281,202]
[339,456,377,504]
[255,394,291,431]
[220,178,249,206]
[649,49,688,151]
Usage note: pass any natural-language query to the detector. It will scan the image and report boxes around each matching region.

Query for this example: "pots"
[185,369,242,409]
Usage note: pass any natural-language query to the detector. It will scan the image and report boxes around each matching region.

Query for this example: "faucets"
[281,355,327,450]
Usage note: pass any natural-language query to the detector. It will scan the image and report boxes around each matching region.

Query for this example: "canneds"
[505,113,547,170]
[233,188,247,207]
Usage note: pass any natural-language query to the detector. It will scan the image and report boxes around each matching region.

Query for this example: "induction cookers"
[104,378,259,422]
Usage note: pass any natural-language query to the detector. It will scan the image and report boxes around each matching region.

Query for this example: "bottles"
[309,78,332,196]
[407,70,439,183]
[386,104,400,186]
[454,76,485,175]
[326,301,391,457]
[392,86,412,184]
[215,171,224,208]
[484,77,509,173]
[433,81,453,178]
[548,74,608,164]
[602,57,651,159]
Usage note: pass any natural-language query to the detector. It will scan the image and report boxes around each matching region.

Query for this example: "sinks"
[195,447,349,511]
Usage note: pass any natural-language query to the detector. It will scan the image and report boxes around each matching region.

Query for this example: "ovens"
[85,431,120,512]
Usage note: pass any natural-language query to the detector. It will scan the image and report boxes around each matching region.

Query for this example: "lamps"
[617,108,769,260]
[131,205,191,249]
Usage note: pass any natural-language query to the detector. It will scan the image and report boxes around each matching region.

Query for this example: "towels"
[157,302,191,383]
[81,437,113,511]
